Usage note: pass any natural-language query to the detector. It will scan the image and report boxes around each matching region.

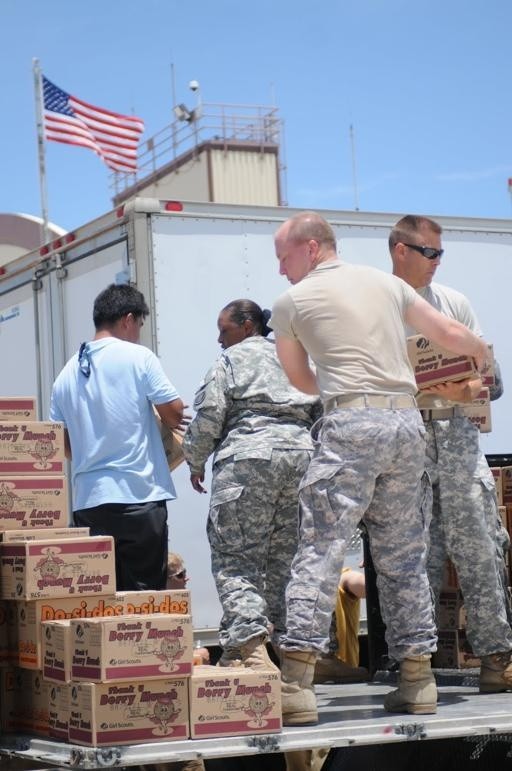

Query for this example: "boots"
[381,650,438,716]
[273,648,322,725]
[476,652,512,696]
[238,633,281,679]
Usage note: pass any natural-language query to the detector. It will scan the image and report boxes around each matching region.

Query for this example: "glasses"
[393,240,444,263]
[76,341,92,378]
[166,567,188,582]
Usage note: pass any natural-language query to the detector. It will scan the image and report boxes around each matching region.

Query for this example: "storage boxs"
[431,466,512,668]
[407,335,494,433]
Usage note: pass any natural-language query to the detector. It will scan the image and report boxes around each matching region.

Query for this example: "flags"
[41,74,142,175]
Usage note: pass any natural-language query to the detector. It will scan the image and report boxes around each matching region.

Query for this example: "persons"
[183,300,315,674]
[260,211,487,727]
[166,553,209,661]
[212,613,370,684]
[367,215,512,695]
[43,284,191,590]
[333,561,369,667]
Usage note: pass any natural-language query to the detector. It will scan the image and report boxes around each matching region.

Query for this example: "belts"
[320,391,416,414]
[421,407,466,421]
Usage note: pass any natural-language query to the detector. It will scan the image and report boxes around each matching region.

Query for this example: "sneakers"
[215,645,244,671]
[313,652,367,686]
